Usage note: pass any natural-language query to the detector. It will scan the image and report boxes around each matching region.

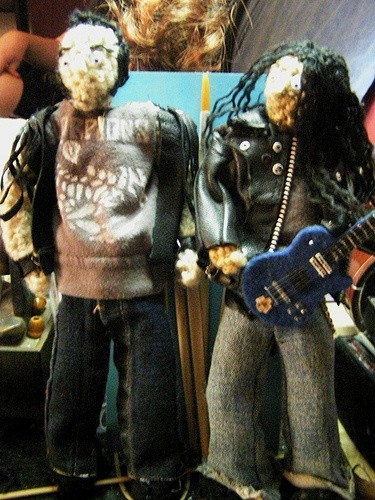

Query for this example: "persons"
[1,8,18,115]
[0,1,251,79]
[188,41,373,500]
[1,10,205,500]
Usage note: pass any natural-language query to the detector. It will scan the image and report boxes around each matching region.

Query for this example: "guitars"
[240,210,375,330]
